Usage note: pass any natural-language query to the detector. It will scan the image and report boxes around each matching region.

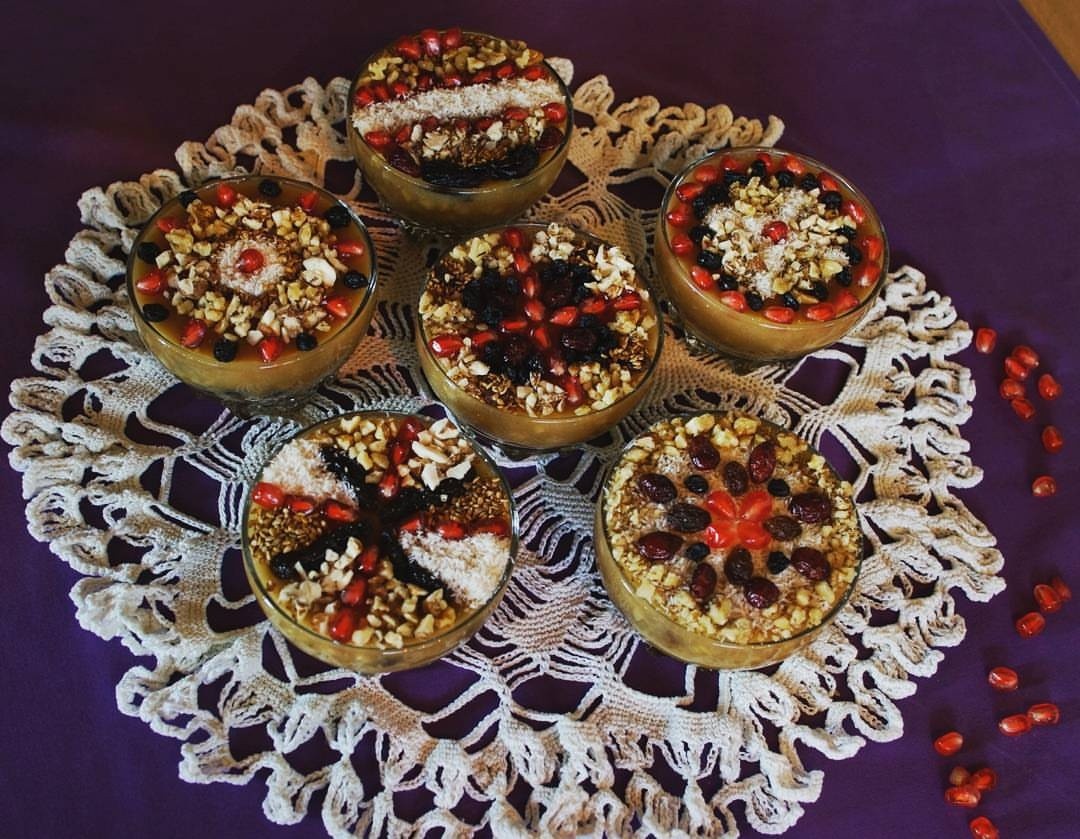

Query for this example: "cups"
[239,408,520,677]
[352,29,576,236]
[124,175,379,411]
[414,222,665,452]
[655,147,891,365]
[594,408,863,671]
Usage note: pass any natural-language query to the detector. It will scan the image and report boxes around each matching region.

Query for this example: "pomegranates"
[135,29,882,642]
[934,328,1072,839]
